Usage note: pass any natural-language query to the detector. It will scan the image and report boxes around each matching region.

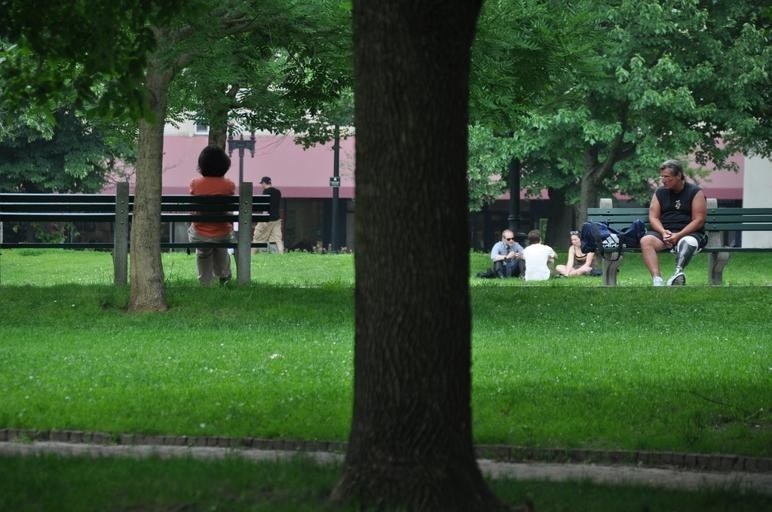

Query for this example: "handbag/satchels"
[580,218,645,261]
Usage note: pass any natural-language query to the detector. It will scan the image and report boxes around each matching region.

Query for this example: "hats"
[260,177,272,185]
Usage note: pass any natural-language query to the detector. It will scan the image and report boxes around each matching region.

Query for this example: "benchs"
[0,181,272,288]
[586,198,772,287]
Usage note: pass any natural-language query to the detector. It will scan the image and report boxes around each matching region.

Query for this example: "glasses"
[506,237,516,242]
[658,175,674,182]
[570,230,579,235]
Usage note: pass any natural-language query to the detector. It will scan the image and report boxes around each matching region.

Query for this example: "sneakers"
[653,273,686,286]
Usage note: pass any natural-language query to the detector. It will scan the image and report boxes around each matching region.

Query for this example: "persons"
[517,228,559,282]
[556,228,597,278]
[250,176,285,254]
[640,160,708,287]
[490,228,525,280]
[187,144,236,286]
